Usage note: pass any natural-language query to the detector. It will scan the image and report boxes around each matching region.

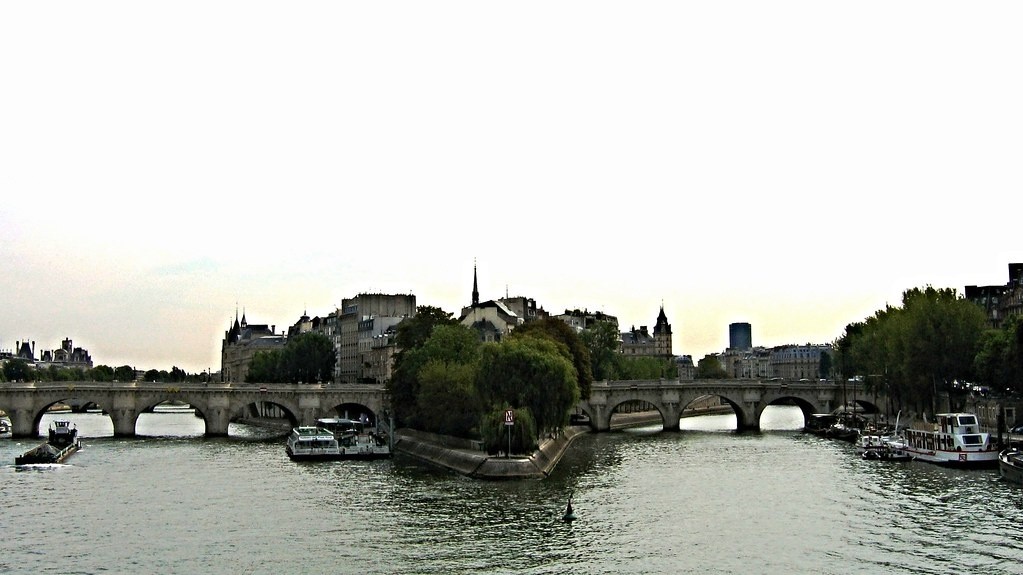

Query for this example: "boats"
[805,411,866,438]
[906,413,999,462]
[0,419,12,433]
[855,410,912,458]
[315,418,391,455]
[15,420,82,464]
[1000,432,1023,484]
[285,424,340,456]
